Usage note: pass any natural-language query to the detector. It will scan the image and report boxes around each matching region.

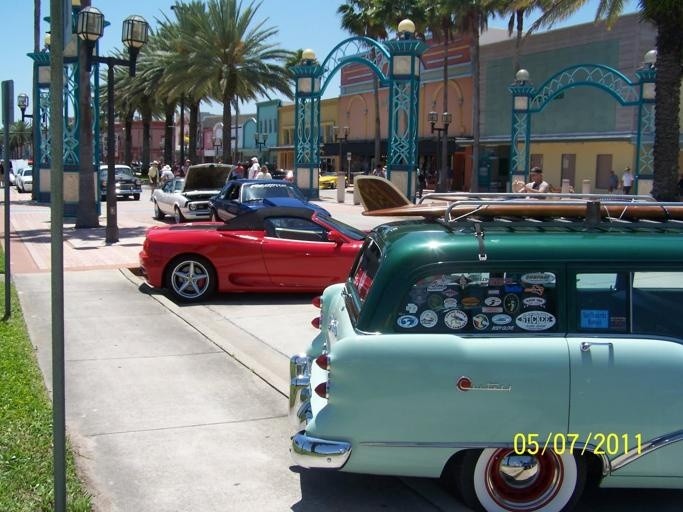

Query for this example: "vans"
[287,174,683,512]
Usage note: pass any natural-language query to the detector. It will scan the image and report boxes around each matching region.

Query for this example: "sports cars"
[138,206,368,303]
[207,179,331,222]
[152,164,235,224]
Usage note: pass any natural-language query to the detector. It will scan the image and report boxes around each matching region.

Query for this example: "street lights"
[16,93,46,123]
[254,133,268,167]
[331,126,352,204]
[386,17,423,204]
[505,69,536,193]
[16,91,50,164]
[332,125,350,171]
[510,68,532,195]
[27,31,69,203]
[211,136,222,155]
[51,1,101,220]
[385,17,432,203]
[253,132,268,169]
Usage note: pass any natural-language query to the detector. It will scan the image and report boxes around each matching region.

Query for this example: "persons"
[259,165,273,180]
[620,166,635,193]
[372,163,384,179]
[1,156,17,189]
[234,161,245,178]
[417,170,426,199]
[181,159,193,178]
[247,156,260,179]
[148,160,160,202]
[513,165,550,193]
[161,165,175,187]
[606,169,619,192]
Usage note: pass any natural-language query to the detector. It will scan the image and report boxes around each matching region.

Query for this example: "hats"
[150,161,160,166]
[185,159,193,163]
[161,166,172,171]
[624,166,631,171]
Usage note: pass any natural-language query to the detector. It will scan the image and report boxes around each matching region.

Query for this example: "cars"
[15,165,33,192]
[100,165,142,201]
